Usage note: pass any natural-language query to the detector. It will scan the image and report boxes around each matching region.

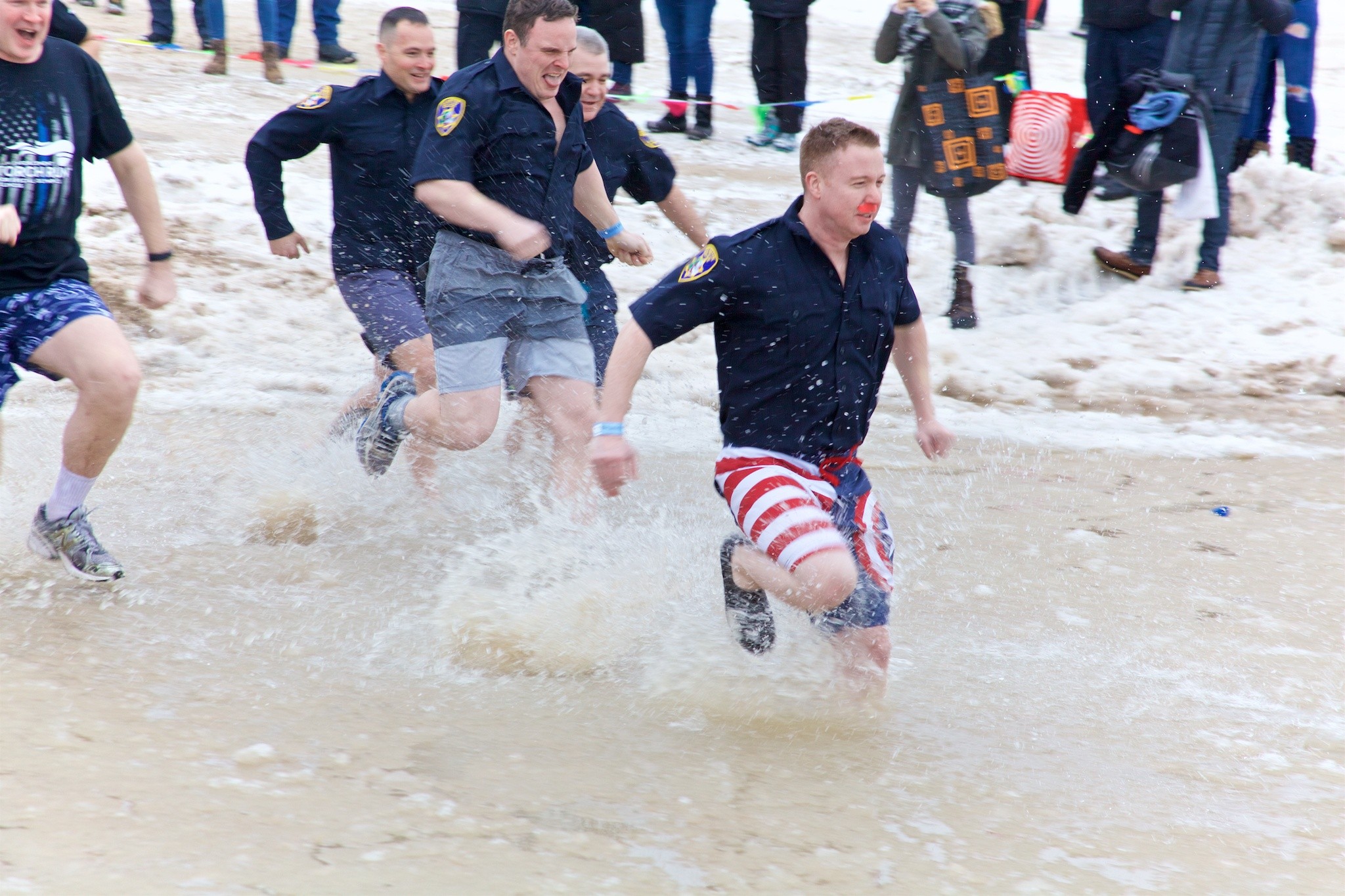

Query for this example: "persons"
[744,0,814,152]
[572,0,643,103]
[647,0,716,140]
[504,26,710,463]
[245,6,449,493]
[589,118,951,691]
[1082,0,1173,201]
[456,0,509,70]
[48,0,101,59]
[1249,0,1318,170]
[876,0,1000,329]
[358,0,654,494]
[1093,0,1295,291]
[0,0,178,582]
[140,0,358,84]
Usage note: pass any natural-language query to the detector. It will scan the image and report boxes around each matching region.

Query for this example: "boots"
[206,39,227,75]
[1287,137,1317,169]
[647,91,689,133]
[1249,141,1269,158]
[262,41,285,84]
[690,93,715,139]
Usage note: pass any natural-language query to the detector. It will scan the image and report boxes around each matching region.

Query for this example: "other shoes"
[950,266,978,327]
[148,31,171,44]
[77,0,94,6]
[202,38,212,50]
[358,370,418,476]
[108,0,124,14]
[718,538,776,655]
[319,43,357,64]
[748,122,780,146]
[277,45,288,60]
[607,82,631,103]
[773,129,796,151]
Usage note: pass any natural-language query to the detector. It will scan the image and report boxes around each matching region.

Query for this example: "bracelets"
[592,423,624,436]
[150,252,172,261]
[595,221,623,239]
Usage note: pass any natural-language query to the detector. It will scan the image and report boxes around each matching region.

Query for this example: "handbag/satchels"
[1007,88,1092,182]
[915,46,1007,198]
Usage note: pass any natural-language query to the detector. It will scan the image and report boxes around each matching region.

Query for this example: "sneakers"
[1183,268,1220,290]
[1093,246,1151,281]
[28,499,124,583]
